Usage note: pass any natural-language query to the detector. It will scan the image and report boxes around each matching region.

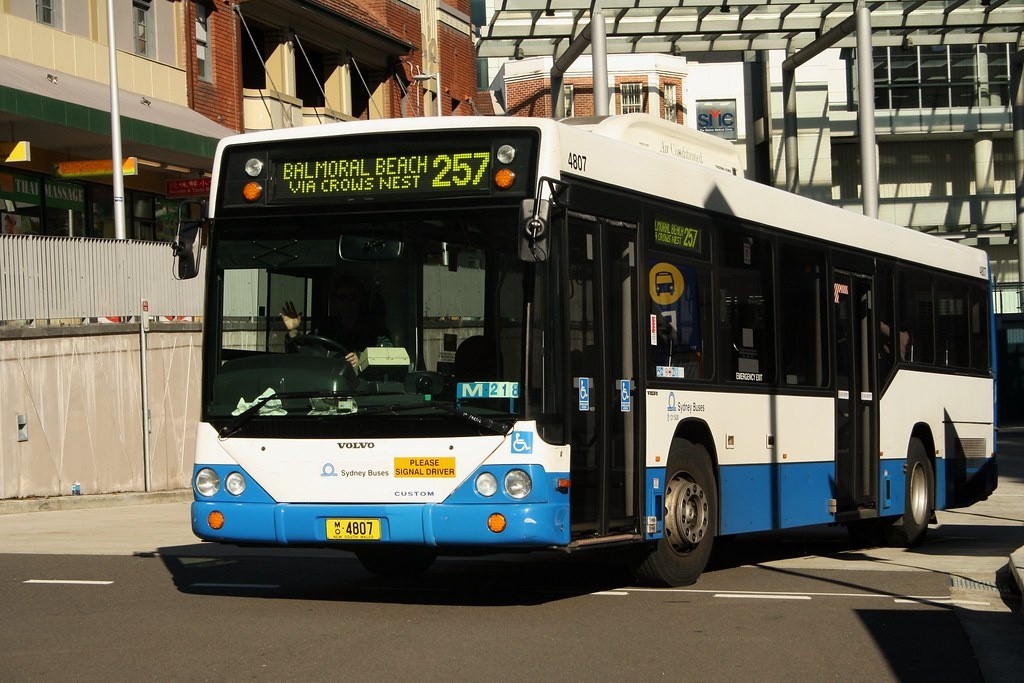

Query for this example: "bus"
[172,109,995,592]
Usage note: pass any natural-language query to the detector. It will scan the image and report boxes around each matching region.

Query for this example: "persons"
[281,257,392,378]
